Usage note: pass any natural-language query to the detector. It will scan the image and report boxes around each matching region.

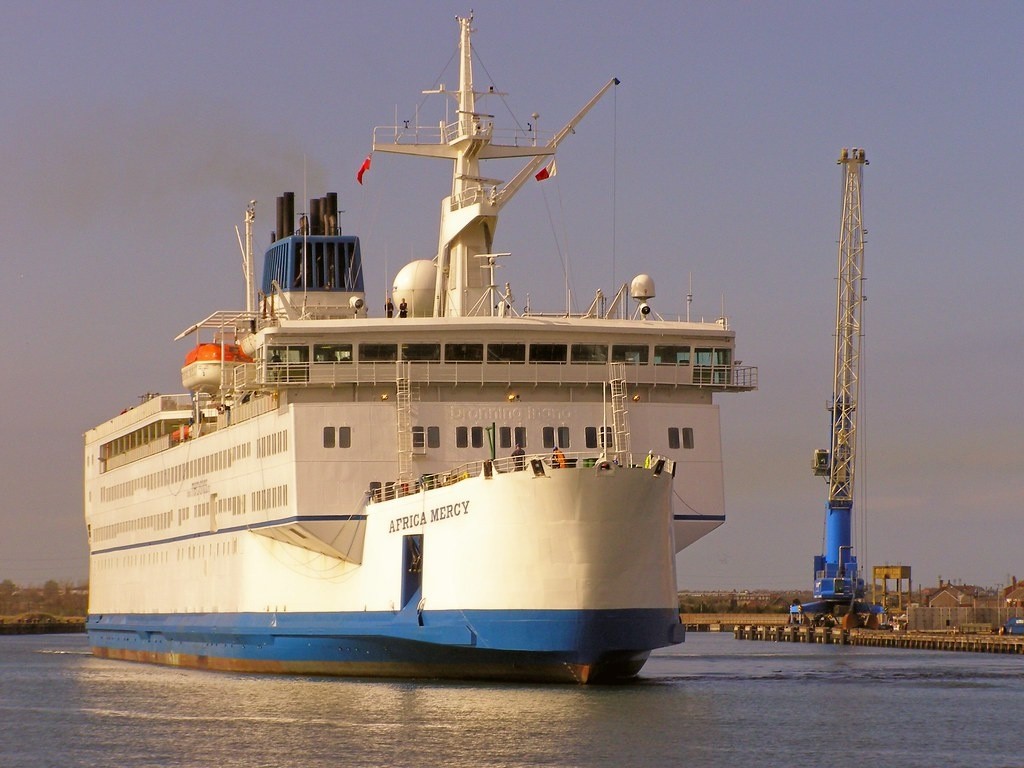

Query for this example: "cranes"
[791,145,888,628]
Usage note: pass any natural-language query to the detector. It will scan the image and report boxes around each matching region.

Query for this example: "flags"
[535,155,557,181]
[356,153,372,186]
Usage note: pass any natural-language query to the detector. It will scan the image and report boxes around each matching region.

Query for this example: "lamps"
[640,303,651,315]
[349,296,364,310]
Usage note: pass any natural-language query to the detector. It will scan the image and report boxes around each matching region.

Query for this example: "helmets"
[553,447,558,450]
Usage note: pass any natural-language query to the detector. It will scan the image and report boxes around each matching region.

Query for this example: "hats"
[516,443,521,446]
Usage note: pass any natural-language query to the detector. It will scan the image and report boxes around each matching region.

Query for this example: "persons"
[270,349,282,362]
[553,446,565,468]
[511,443,525,470]
[399,297,408,317]
[384,298,394,318]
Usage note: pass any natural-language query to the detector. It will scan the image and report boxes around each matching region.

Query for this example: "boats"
[179,342,252,395]
[173,425,204,441]
[80,6,761,685]
